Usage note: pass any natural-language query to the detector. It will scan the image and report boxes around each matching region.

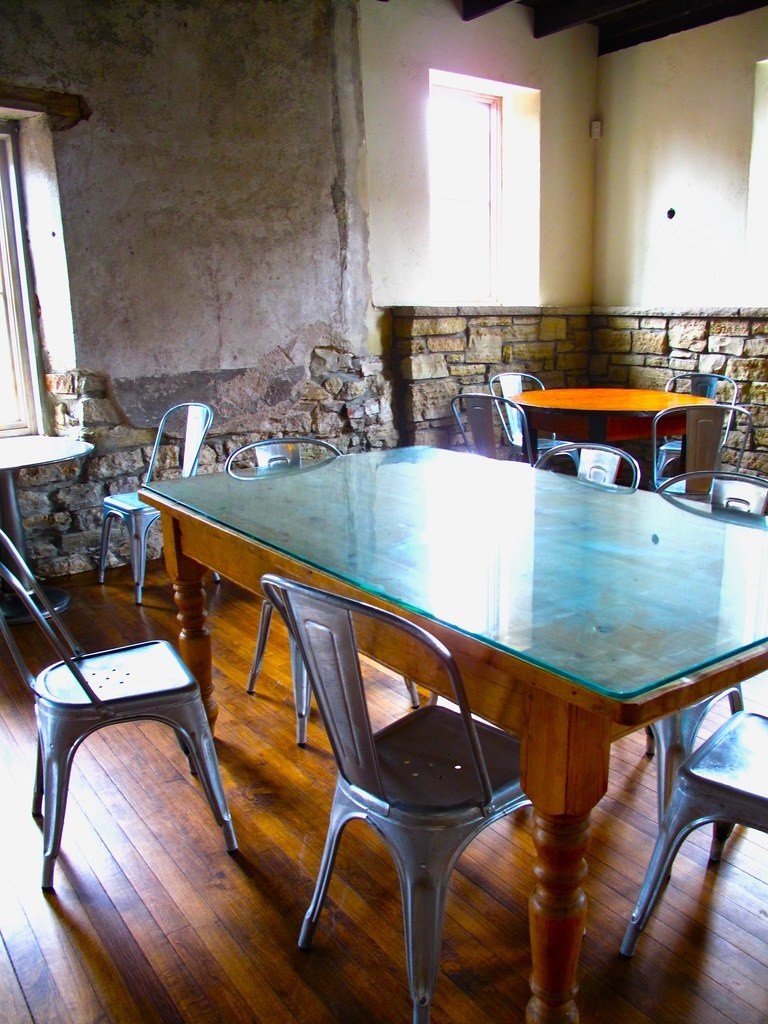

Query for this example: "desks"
[139,447,766,1023]
[508,387,717,444]
[0,435,96,624]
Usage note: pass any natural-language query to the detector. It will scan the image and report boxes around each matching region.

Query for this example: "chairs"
[656,373,736,475]
[261,572,533,1024]
[618,709,768,958]
[651,404,752,495]
[451,392,533,464]
[646,471,768,832]
[97,402,221,605]
[489,373,544,446]
[225,437,420,748]
[533,443,641,489]
[0,528,238,891]
